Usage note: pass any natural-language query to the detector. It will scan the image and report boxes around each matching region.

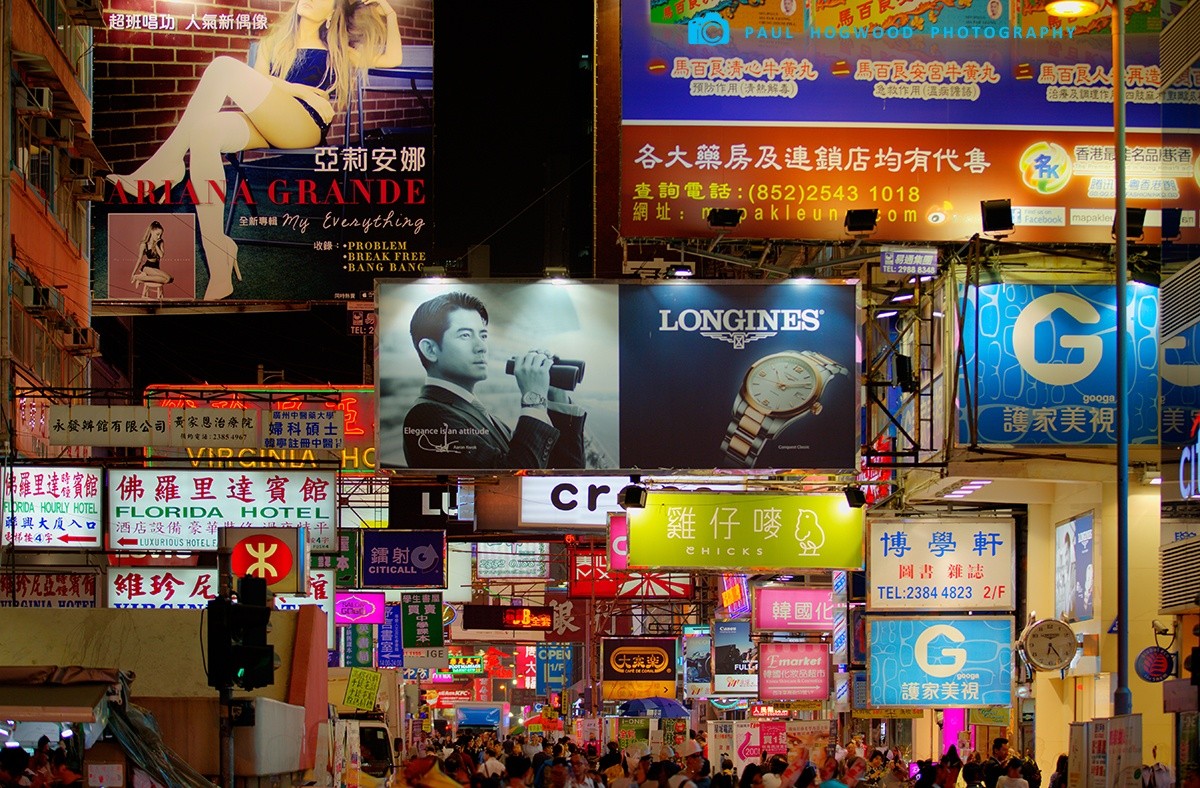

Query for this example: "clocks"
[1016,610,1099,682]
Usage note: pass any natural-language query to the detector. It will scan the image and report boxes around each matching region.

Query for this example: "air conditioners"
[19,283,99,358]
[15,83,106,205]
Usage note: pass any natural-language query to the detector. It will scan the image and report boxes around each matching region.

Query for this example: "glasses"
[569,761,586,767]
[690,751,704,760]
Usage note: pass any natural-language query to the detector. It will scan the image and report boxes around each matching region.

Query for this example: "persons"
[129,220,176,289]
[1059,532,1077,618]
[0,747,35,787]
[417,727,1066,788]
[54,737,71,777]
[33,736,54,781]
[103,0,405,302]
[399,291,588,470]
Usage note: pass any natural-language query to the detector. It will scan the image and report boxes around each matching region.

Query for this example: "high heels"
[105,158,186,209]
[131,268,148,284]
[196,235,244,306]
[135,274,145,289]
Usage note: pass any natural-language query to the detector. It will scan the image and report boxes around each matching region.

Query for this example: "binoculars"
[505,355,586,391]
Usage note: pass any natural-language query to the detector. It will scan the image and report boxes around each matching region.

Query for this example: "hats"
[674,739,702,757]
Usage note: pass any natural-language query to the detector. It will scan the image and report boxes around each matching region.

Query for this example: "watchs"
[719,349,851,469]
[520,392,549,409]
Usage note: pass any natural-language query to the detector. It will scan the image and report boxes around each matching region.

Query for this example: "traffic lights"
[231,574,277,692]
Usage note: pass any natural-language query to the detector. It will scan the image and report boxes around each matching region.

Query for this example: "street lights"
[1046,0,1130,716]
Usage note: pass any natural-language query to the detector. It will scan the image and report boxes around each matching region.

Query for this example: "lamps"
[841,204,879,243]
[1142,461,1161,486]
[977,196,1016,246]
[623,474,649,510]
[1155,206,1187,249]
[872,268,943,321]
[1110,205,1147,249]
[706,205,743,231]
[663,264,692,280]
[893,352,922,396]
[788,267,817,281]
[843,481,866,509]
